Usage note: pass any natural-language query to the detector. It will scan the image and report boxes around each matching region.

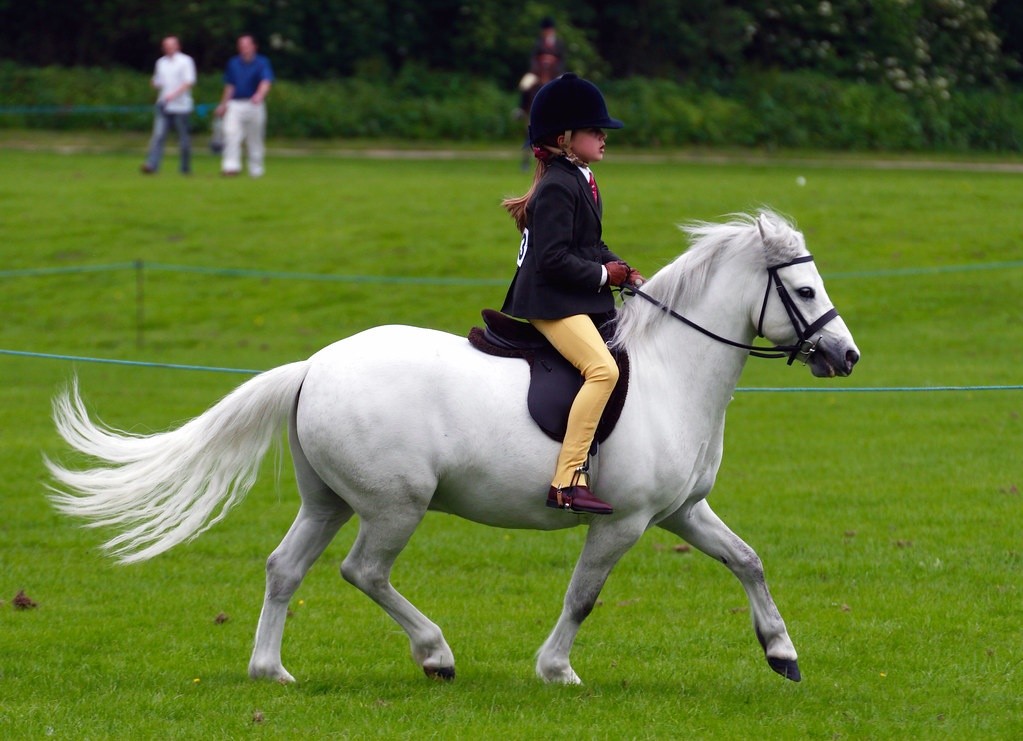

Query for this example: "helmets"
[530,71,624,145]
[540,18,554,30]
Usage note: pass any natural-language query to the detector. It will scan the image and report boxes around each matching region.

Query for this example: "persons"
[500,71,647,515]
[528,17,568,78]
[137,37,197,176]
[217,34,274,179]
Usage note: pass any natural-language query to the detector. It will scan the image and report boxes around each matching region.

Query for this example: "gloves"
[629,268,645,286]
[604,260,630,286]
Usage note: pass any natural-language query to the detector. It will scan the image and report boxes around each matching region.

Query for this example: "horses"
[42,205,864,689]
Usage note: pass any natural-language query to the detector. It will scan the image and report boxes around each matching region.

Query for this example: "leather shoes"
[546,486,613,515]
